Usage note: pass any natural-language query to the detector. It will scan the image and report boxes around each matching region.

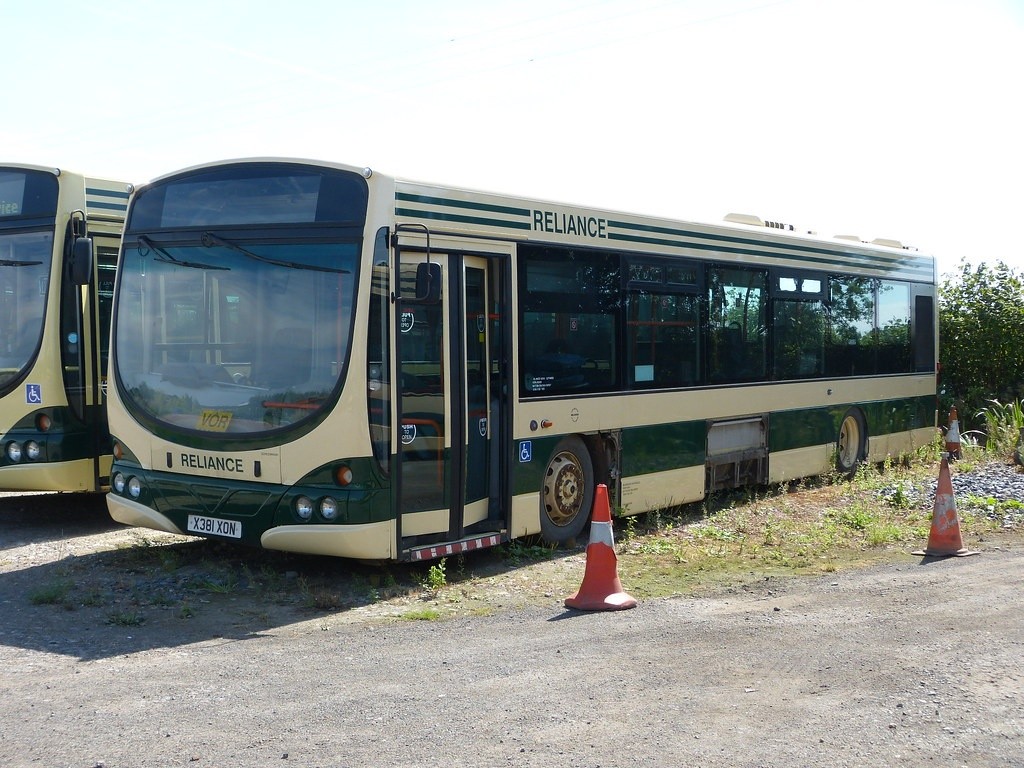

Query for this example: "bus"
[0,160,134,494]
[102,156,942,570]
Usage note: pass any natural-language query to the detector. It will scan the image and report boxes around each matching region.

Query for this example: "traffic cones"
[910,452,983,558]
[563,483,638,612]
[944,403,962,465]
